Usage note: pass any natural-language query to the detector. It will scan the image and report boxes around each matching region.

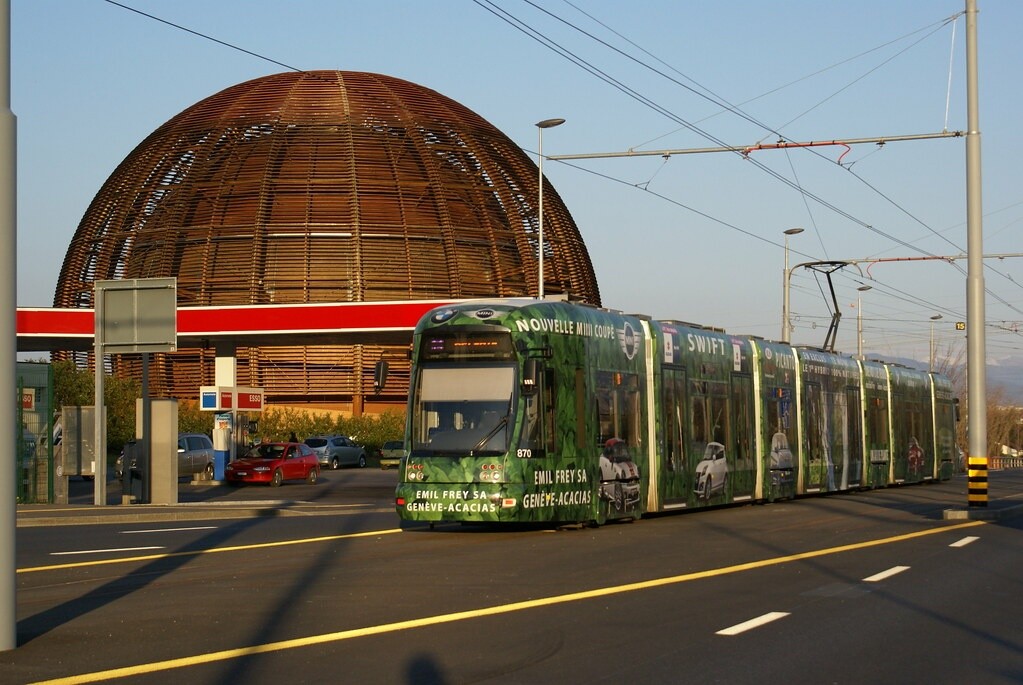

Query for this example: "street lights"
[930,314,943,374]
[781,228,805,343]
[534,118,566,301]
[857,286,872,356]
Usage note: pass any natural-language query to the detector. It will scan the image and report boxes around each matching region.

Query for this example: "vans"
[18,423,39,482]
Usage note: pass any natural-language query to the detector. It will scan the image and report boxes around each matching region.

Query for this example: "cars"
[379,440,404,471]
[225,442,320,489]
[954,443,966,472]
[115,434,214,486]
[305,436,367,470]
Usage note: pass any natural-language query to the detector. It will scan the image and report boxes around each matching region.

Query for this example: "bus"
[375,299,960,524]
